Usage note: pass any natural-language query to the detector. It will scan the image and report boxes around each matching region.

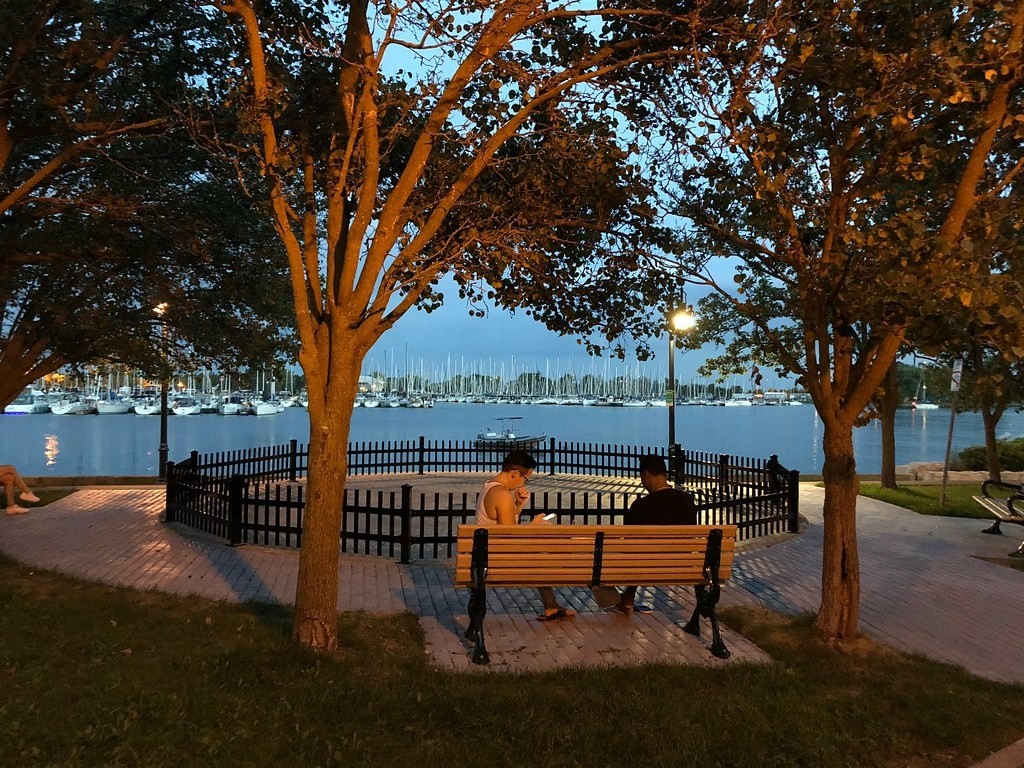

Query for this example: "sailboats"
[4,341,804,416]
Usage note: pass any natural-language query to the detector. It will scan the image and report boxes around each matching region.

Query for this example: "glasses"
[513,468,529,484]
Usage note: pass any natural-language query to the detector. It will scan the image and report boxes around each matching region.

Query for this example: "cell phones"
[543,514,557,521]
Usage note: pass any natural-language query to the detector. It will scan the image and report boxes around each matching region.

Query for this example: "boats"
[913,401,938,410]
[473,417,547,452]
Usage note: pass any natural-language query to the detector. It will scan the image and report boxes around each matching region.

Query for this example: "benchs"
[970,480,1024,559]
[446,525,737,665]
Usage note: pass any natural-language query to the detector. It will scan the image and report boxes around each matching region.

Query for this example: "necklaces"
[655,487,671,491]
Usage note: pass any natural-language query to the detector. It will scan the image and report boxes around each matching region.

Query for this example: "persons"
[610,455,697,615]
[474,451,577,620]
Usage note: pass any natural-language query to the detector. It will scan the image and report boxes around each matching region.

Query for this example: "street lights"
[152,303,171,483]
[668,299,696,483]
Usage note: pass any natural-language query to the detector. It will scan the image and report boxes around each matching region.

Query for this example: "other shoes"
[614,594,633,614]
[634,600,652,613]
[537,606,575,623]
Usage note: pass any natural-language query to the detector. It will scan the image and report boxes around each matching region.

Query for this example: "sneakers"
[6,505,29,515]
[19,491,40,502]
